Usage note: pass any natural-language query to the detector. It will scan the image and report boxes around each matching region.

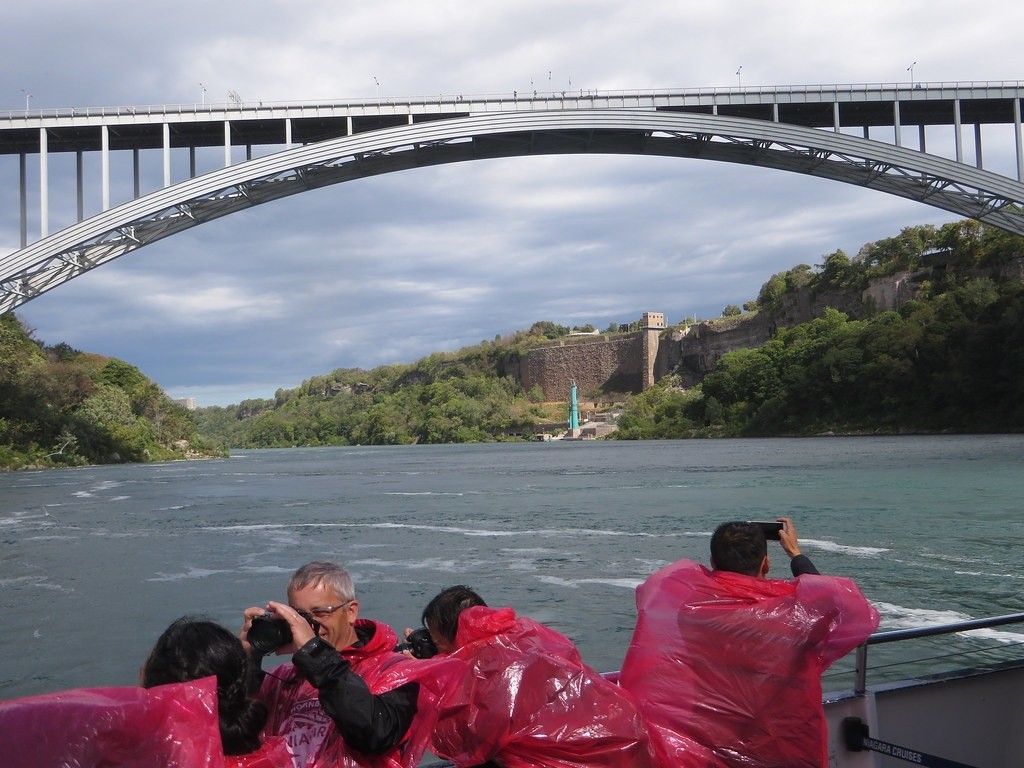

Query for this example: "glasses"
[296,600,351,624]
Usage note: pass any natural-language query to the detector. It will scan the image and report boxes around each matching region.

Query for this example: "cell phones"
[748,520,784,541]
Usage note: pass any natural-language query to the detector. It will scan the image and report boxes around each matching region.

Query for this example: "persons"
[396,584,757,768]
[0,616,270,768]
[239,560,420,768]
[659,517,843,768]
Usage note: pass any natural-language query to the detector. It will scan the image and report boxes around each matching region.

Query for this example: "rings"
[291,613,298,619]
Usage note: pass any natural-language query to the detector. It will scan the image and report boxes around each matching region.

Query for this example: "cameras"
[247,609,321,654]
[394,626,438,660]
